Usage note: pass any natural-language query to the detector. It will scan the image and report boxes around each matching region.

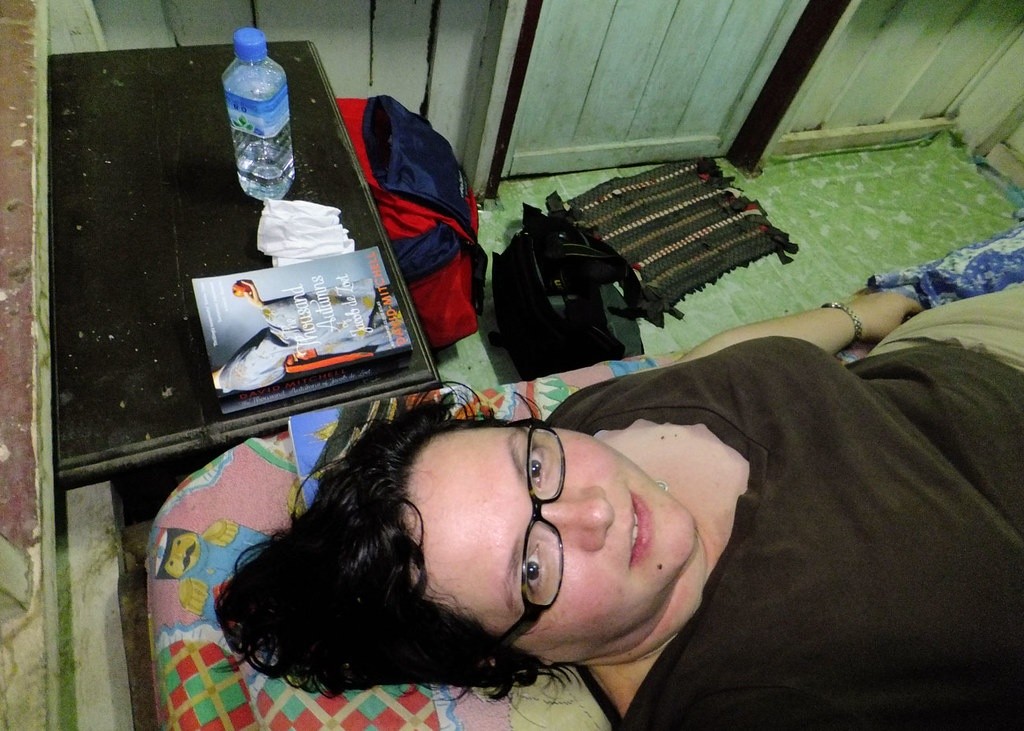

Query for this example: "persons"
[213,277,410,392]
[218,282,1024,731]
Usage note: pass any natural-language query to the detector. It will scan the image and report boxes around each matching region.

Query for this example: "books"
[190,246,413,416]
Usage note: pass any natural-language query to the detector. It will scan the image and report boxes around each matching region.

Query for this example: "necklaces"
[656,479,667,491]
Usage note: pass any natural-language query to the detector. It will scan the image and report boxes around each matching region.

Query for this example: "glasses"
[497,417,566,650]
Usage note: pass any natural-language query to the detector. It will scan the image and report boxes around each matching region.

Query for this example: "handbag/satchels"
[488,191,649,381]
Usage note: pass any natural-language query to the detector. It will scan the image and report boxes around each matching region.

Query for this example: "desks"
[46,40,443,522]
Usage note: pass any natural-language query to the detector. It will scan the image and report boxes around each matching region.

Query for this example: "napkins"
[257,194,355,267]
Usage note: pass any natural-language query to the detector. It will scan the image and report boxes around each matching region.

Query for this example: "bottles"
[222,27,296,203]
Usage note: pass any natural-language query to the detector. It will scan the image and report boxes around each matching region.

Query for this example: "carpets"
[545,157,798,328]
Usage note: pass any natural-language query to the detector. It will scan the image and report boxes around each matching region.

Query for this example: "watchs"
[821,302,862,348]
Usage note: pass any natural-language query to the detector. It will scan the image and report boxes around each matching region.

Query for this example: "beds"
[491,219,1024,429]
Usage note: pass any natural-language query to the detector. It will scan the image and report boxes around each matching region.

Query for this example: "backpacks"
[337,95,488,353]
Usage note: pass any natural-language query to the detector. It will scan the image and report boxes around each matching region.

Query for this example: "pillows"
[148,432,612,731]
